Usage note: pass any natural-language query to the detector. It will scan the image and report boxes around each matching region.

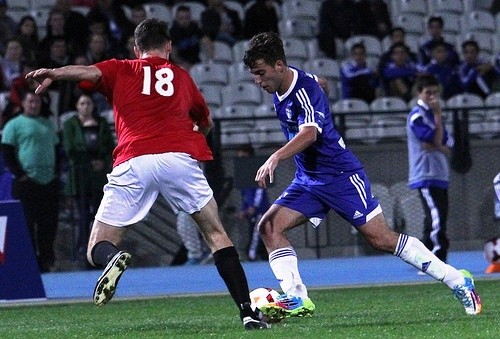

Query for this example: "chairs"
[351,181,429,259]
[6,0,500,147]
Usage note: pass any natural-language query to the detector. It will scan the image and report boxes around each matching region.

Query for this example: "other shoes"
[93,250,132,307]
[240,306,268,329]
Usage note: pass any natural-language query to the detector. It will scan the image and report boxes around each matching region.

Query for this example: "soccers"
[250,287,285,323]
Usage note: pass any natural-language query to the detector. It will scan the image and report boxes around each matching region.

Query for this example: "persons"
[243,31,482,317]
[63,93,116,253]
[0,0,500,128]
[236,139,273,261]
[2,90,60,274]
[25,18,272,330]
[407,77,454,276]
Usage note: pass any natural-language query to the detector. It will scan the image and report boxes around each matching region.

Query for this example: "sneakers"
[261,293,315,319]
[451,270,481,315]
